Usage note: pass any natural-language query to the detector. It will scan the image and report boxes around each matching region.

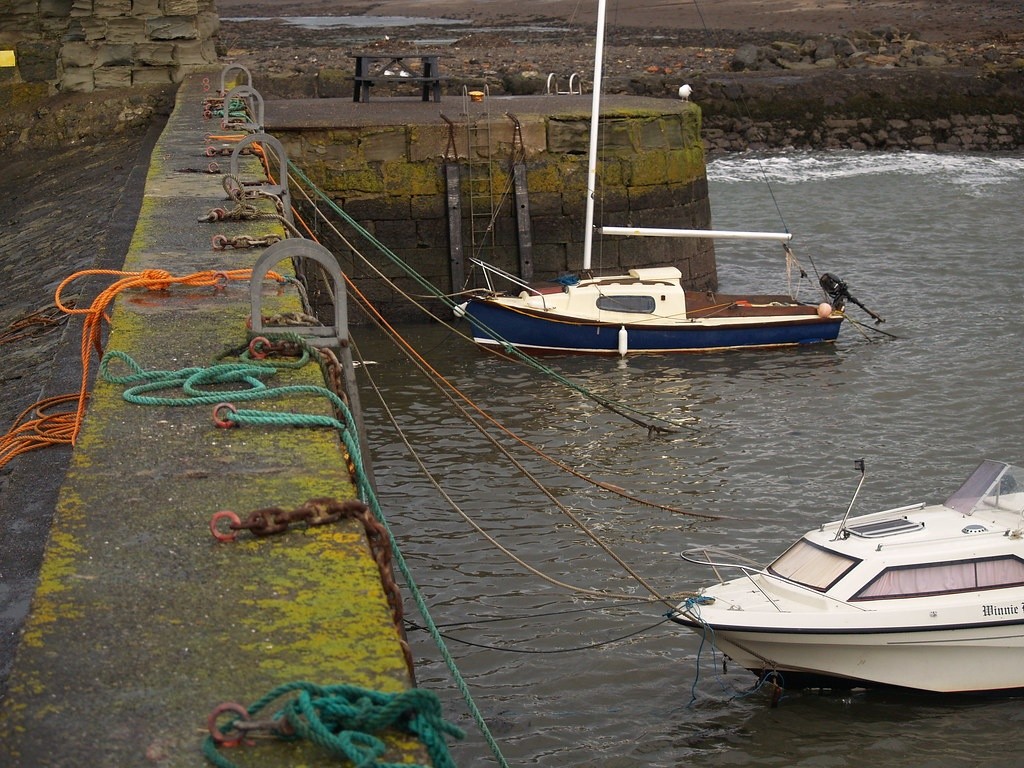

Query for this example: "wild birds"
[679,84,693,103]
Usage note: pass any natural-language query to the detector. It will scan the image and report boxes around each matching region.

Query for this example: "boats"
[668,458,1024,705]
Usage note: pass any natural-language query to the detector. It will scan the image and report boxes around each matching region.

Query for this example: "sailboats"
[447,0,881,351]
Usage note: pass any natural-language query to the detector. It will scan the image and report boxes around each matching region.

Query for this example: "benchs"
[353,76,450,83]
[345,76,353,80]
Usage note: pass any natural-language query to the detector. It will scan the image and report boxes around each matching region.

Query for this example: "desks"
[346,50,445,103]
